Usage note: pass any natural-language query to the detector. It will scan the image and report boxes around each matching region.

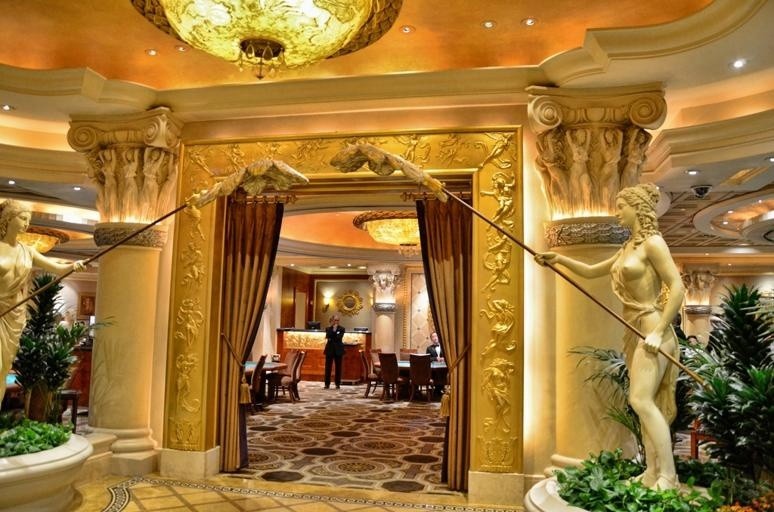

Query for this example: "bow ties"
[434,344,439,348]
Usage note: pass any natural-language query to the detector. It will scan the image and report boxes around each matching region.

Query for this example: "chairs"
[358,347,436,402]
[241,351,306,415]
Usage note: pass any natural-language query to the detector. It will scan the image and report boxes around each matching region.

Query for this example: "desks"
[372,360,447,397]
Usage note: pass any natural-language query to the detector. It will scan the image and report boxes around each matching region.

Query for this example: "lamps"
[131,1,405,79]
[352,209,424,247]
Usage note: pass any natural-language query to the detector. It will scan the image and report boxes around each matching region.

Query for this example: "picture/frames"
[76,291,96,320]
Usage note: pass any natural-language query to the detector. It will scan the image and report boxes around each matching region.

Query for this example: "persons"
[534,183,685,492]
[372,275,395,304]
[0,198,91,410]
[323,315,345,389]
[426,332,449,395]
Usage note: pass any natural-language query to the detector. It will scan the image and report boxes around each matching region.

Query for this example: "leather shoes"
[324,385,340,389]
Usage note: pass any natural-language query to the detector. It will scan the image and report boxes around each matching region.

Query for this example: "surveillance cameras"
[691,186,713,198]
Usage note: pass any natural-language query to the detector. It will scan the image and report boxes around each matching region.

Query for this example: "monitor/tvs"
[306,321,321,329]
[354,327,368,331]
